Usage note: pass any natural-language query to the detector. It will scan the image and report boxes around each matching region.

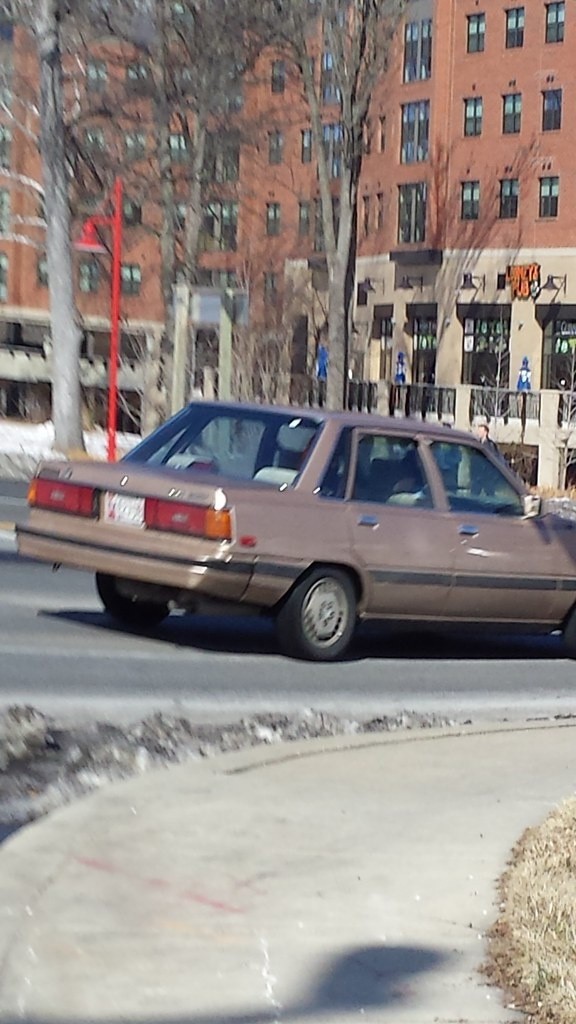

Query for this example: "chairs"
[388,447,468,511]
[254,464,299,491]
[165,452,219,475]
[340,432,386,497]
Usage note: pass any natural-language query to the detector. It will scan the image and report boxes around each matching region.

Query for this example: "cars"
[15,400,576,663]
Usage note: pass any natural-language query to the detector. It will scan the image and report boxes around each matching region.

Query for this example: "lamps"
[396,275,423,293]
[459,273,486,291]
[359,275,385,296]
[539,274,568,294]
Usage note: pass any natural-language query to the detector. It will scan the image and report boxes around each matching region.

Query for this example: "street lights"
[74,176,123,465]
